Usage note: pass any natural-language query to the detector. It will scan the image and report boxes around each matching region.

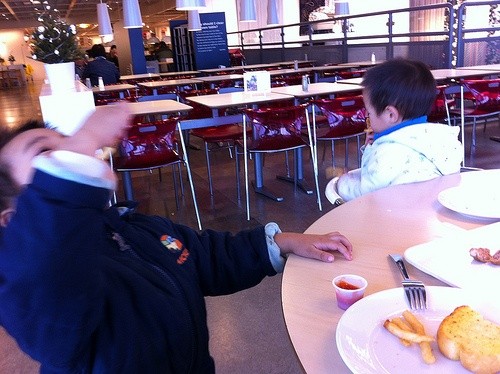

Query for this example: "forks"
[389,253,427,310]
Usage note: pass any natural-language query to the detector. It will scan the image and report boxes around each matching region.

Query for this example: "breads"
[437,305,500,374]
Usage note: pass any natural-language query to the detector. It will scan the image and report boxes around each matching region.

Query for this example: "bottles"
[294,60,298,70]
[371,52,376,62]
[302,75,309,92]
[98,77,105,91]
[86,78,92,90]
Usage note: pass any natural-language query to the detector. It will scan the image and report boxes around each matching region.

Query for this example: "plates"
[438,186,500,220]
[335,286,500,374]
[403,221,500,288]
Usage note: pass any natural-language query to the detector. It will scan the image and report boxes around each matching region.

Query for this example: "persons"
[0,98,353,374]
[83,44,120,89]
[326,58,466,210]
[155,41,174,59]
[106,45,119,67]
[153,43,160,60]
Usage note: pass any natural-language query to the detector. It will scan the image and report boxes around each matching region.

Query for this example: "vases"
[44,62,76,90]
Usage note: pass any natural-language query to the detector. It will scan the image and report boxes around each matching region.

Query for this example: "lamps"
[96,0,113,36]
[267,0,279,25]
[335,3,349,15]
[240,0,257,22]
[176,0,206,10]
[187,11,201,31]
[122,0,143,28]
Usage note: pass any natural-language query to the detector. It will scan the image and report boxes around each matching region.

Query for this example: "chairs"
[109,79,500,231]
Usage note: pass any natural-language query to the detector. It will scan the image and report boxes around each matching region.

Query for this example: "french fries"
[383,310,435,363]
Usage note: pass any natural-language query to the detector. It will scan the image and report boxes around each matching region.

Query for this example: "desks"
[281,169,500,374]
[95,60,500,201]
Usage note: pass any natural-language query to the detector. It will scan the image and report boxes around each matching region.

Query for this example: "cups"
[332,275,367,310]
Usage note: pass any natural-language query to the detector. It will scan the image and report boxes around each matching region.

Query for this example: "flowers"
[24,0,87,64]
